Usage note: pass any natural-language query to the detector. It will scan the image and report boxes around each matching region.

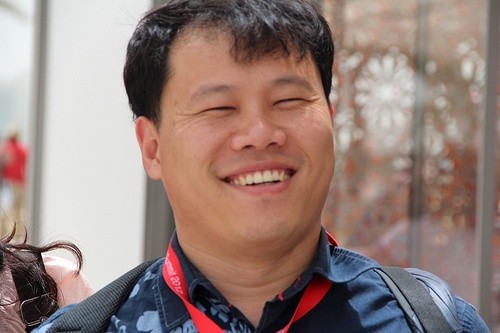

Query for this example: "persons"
[28,0,493,333]
[0,220,95,333]
